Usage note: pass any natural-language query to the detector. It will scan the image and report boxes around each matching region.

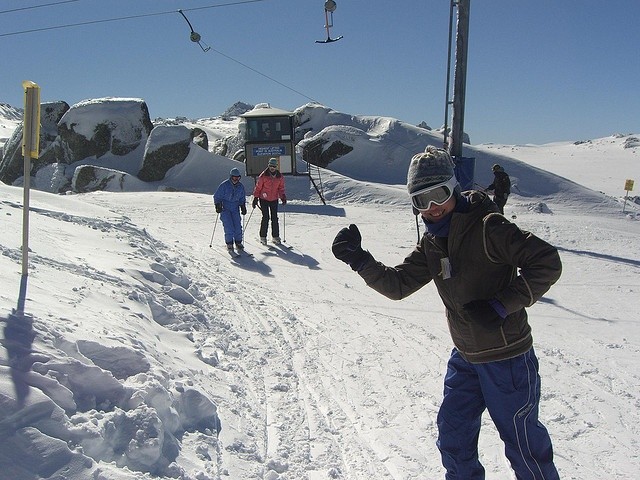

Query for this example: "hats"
[492,164,500,172]
[230,168,240,176]
[406,145,462,214]
[268,157,278,167]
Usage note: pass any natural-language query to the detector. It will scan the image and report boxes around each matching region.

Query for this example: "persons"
[331,146,561,480]
[486,163,510,215]
[253,158,287,245]
[212,168,248,258]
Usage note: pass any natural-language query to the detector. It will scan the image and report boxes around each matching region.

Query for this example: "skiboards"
[228,250,253,258]
[254,237,293,254]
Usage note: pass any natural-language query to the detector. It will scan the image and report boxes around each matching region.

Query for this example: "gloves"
[252,197,258,208]
[461,298,507,331]
[503,192,508,199]
[332,224,368,271]
[215,204,222,214]
[281,199,286,206]
[484,188,490,192]
[240,205,246,216]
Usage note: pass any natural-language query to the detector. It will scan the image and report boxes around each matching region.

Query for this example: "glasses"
[408,174,458,212]
[230,175,241,181]
[268,164,278,169]
[266,131,271,133]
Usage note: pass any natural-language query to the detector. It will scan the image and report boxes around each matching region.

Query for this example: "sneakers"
[235,243,244,249]
[227,244,234,251]
[272,236,281,243]
[259,236,267,245]
